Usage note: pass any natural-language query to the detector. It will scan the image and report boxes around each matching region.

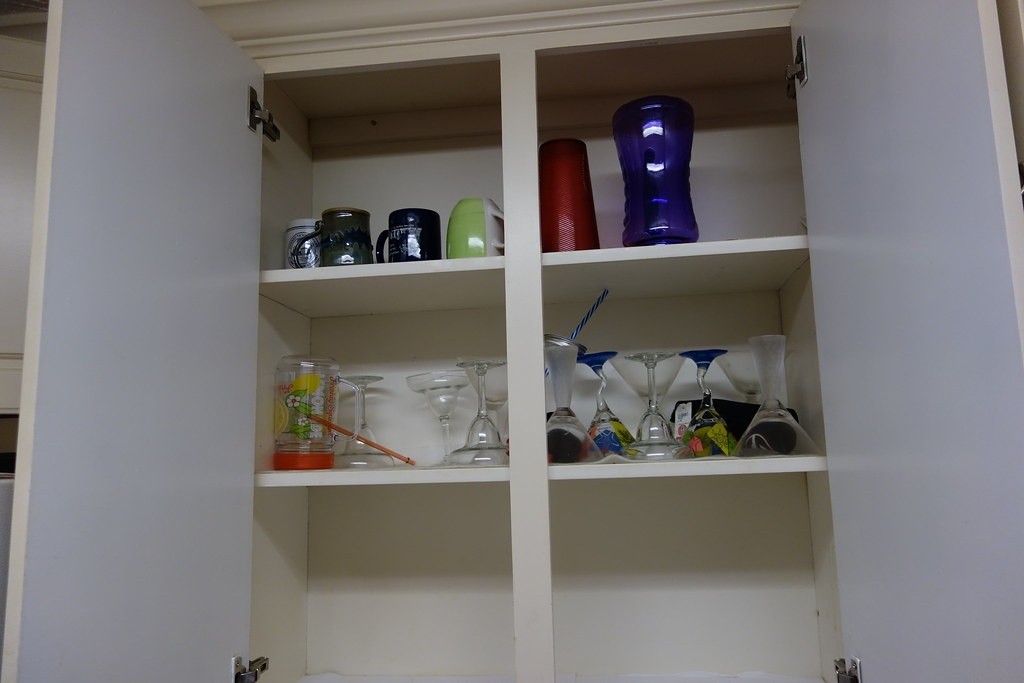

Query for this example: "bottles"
[577,352,635,461]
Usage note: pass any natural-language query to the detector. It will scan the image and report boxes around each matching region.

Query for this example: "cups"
[316,207,374,267]
[376,208,441,263]
[274,353,362,471]
[285,219,321,268]
[446,197,504,260]
[612,94,699,247]
[539,138,600,253]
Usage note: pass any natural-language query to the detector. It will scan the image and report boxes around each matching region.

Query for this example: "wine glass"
[715,335,823,458]
[545,346,605,465]
[609,334,680,461]
[448,355,509,467]
[334,375,394,469]
[406,370,472,469]
[680,348,738,459]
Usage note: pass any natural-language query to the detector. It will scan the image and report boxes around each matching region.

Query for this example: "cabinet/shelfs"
[0,1,1024,683]
